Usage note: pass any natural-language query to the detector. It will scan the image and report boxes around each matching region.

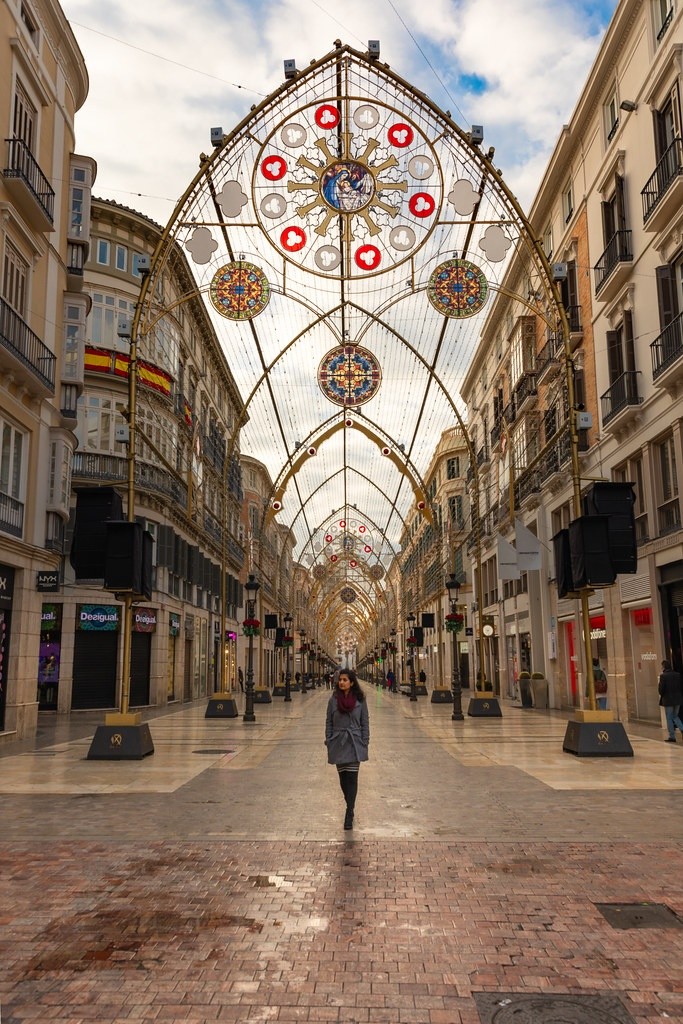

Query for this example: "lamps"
[621,100,636,113]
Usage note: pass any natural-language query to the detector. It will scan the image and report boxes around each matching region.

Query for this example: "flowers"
[390,646,398,655]
[242,619,261,637]
[407,637,417,648]
[444,614,464,635]
[282,637,294,647]
[381,653,387,659]
[300,646,306,653]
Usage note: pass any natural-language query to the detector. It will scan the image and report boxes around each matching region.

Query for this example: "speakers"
[586,482,638,574]
[70,491,122,582]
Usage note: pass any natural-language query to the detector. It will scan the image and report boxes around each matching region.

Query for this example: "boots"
[338,771,358,828]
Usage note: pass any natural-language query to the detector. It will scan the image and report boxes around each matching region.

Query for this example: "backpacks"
[594,672,607,693]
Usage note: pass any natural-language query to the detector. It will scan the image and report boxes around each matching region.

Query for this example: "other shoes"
[664,738,676,742]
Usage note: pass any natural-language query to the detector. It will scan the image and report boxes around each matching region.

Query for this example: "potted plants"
[515,672,548,709]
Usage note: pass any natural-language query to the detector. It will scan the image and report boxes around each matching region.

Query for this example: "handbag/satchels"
[388,678,392,686]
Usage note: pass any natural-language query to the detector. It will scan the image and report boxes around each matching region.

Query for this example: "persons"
[420,670,426,682]
[324,667,340,689]
[351,667,356,672]
[325,669,370,830]
[658,661,683,741]
[387,669,394,692]
[584,658,607,709]
[238,666,244,692]
[477,668,486,680]
[295,672,302,683]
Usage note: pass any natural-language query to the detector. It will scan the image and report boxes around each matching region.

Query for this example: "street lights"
[445,572,465,721]
[243,574,260,721]
[310,638,343,689]
[299,628,308,694]
[380,638,387,689]
[282,612,294,701]
[406,611,418,701]
[355,643,380,687]
[390,629,398,693]
